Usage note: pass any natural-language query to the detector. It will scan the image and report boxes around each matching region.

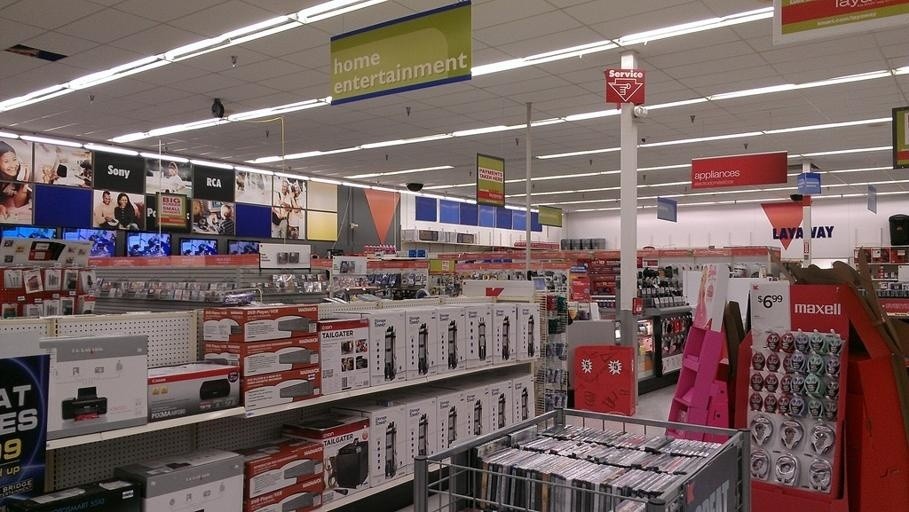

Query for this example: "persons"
[275,180,293,208]
[93,191,141,229]
[272,206,305,239]
[0,140,30,182]
[0,183,29,220]
[214,205,234,234]
[131,244,141,255]
[115,193,140,230]
[184,249,193,256]
[291,183,306,209]
[197,249,205,255]
[161,162,185,192]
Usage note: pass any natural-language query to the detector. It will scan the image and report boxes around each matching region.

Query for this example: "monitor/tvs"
[0,225,57,244]
[227,239,261,255]
[61,225,117,257]
[124,230,172,257]
[178,237,218,256]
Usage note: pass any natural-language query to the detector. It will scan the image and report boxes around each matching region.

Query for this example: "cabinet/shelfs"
[2,245,909,512]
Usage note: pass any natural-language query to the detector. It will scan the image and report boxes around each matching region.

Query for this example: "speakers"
[889,214,909,246]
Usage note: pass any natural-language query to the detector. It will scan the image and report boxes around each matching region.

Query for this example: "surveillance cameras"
[790,194,804,202]
[407,183,423,192]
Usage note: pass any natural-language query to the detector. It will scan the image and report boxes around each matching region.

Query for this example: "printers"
[62,386,107,419]
[200,379,230,399]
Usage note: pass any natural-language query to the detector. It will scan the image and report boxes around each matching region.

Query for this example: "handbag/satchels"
[287,226,299,239]
[272,212,281,225]
